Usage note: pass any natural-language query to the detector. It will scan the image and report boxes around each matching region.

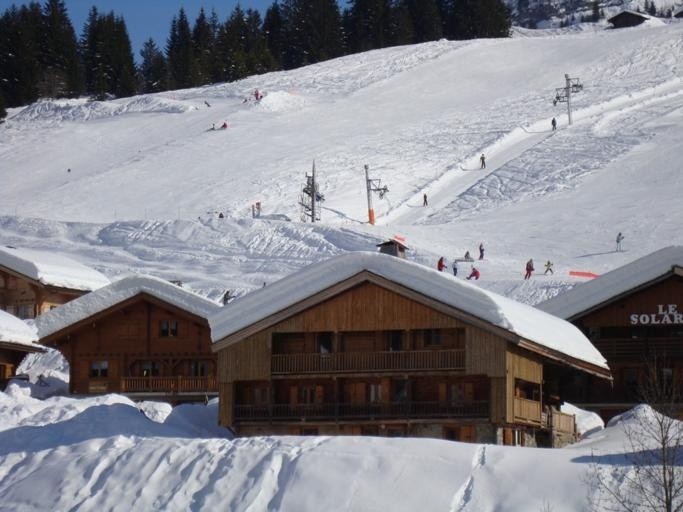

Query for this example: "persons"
[542,260,553,274]
[479,153,487,169]
[222,290,237,305]
[478,242,485,261]
[437,256,447,272]
[464,266,480,281]
[451,260,458,277]
[615,231,624,253]
[523,258,535,280]
[422,192,428,207]
[551,117,557,132]
